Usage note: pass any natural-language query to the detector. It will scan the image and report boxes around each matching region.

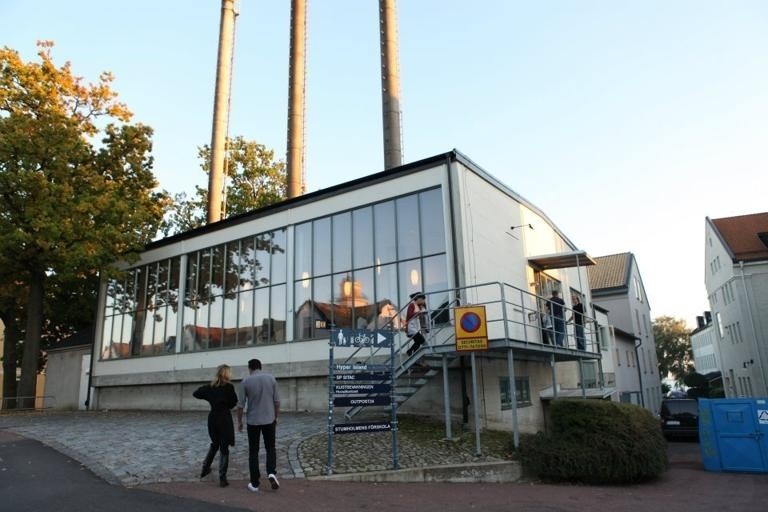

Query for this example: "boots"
[219,453,231,488]
[200,444,219,478]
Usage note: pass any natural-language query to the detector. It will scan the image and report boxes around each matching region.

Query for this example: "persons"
[563,294,587,351]
[544,290,567,347]
[236,359,281,492]
[542,303,556,346]
[405,292,428,357]
[192,364,238,488]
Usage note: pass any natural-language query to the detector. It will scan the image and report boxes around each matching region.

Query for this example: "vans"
[660,398,700,442]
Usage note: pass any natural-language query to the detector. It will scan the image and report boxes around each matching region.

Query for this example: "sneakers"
[248,483,258,492]
[268,474,280,490]
